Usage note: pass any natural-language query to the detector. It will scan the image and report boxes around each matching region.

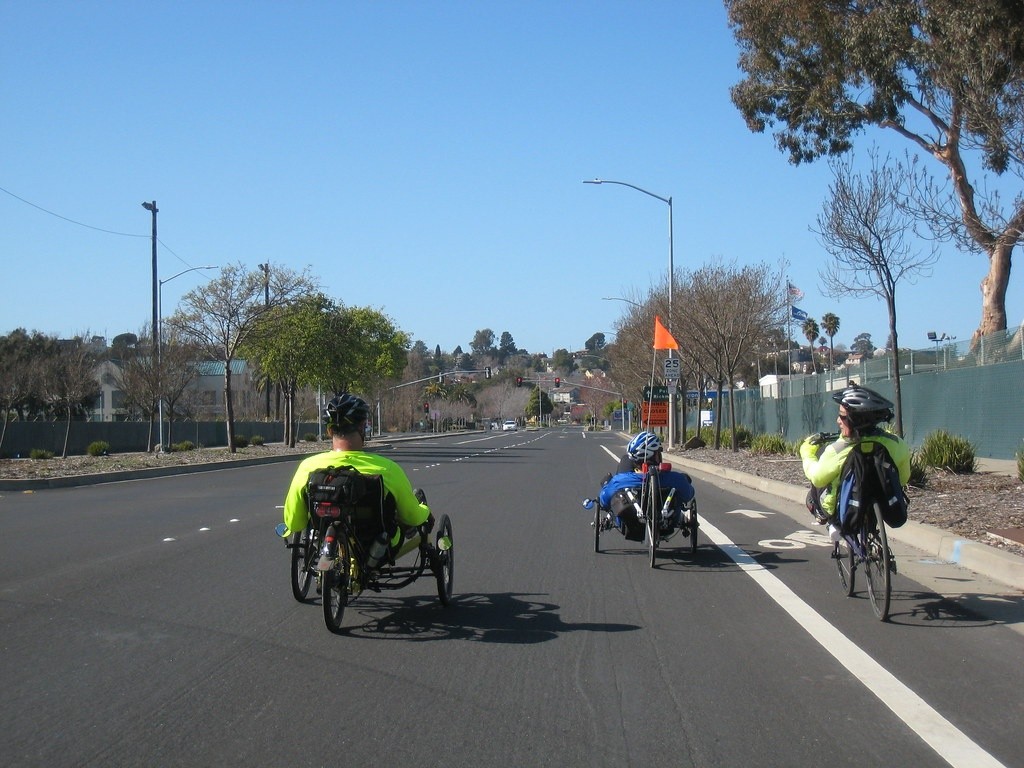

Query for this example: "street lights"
[157,265,219,454]
[581,177,677,454]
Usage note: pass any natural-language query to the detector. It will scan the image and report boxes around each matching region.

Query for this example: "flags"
[788,283,803,302]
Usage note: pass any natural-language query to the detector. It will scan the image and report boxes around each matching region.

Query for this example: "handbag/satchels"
[837,440,909,536]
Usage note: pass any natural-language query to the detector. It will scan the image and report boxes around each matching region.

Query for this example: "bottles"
[364,532,388,573]
[346,537,358,589]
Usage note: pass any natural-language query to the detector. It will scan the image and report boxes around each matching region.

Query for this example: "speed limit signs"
[664,357,681,379]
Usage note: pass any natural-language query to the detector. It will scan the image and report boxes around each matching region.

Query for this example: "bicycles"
[808,427,900,625]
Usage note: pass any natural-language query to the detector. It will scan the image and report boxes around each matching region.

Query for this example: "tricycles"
[581,451,701,569]
[275,463,455,631]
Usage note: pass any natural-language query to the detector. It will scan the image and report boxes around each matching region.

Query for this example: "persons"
[600,431,694,528]
[800,387,911,539]
[284,395,430,550]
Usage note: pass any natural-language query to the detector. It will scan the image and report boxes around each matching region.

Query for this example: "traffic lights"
[517,377,522,387]
[623,399,627,409]
[555,378,560,387]
[424,402,429,413]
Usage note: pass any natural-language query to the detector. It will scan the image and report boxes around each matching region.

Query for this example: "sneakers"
[404,487,427,539]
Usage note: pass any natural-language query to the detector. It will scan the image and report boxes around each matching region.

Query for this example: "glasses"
[838,413,849,421]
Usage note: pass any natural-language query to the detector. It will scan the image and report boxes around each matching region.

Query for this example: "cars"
[502,420,517,432]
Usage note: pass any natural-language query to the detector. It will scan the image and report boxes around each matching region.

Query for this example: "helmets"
[321,394,370,426]
[627,431,663,464]
[833,386,895,422]
